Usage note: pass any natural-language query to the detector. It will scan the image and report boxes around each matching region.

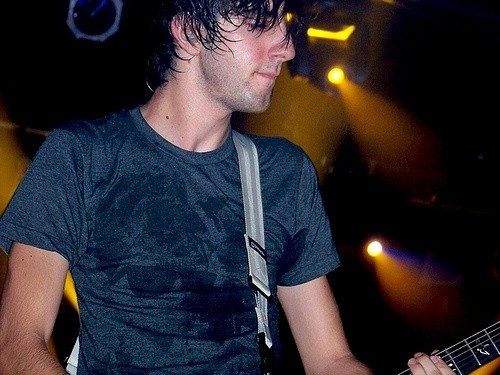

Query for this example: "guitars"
[397,320,500,375]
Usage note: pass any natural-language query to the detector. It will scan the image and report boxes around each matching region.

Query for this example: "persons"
[0,0,456,375]
